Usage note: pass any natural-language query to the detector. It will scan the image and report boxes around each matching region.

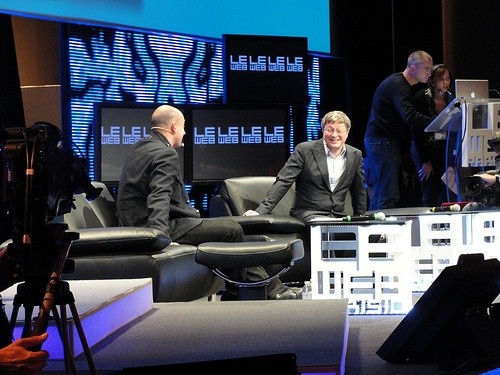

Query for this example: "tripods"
[9,223,97,375]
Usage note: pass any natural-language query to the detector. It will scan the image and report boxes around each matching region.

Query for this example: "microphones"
[343,212,385,222]
[182,143,185,147]
[430,204,460,212]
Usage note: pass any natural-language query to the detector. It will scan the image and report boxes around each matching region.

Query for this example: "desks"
[301,209,500,309]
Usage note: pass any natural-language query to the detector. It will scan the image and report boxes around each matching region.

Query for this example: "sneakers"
[275,287,303,300]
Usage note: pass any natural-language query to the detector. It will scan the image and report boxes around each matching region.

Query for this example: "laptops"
[455,79,489,100]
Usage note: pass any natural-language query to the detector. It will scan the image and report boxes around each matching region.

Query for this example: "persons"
[242,114,368,282]
[364,51,500,209]
[118,105,303,301]
[0,296,50,375]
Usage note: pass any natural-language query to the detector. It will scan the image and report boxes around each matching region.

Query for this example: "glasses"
[324,128,346,134]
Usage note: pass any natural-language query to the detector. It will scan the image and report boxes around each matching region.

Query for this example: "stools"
[194,239,304,301]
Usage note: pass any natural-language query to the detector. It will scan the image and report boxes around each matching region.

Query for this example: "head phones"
[427,64,447,87]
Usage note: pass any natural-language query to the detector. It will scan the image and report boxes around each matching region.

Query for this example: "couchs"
[64,176,381,302]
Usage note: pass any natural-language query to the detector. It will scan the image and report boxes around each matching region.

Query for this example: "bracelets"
[495,174,500,183]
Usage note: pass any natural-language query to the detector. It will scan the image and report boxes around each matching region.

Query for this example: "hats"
[431,64,446,72]
